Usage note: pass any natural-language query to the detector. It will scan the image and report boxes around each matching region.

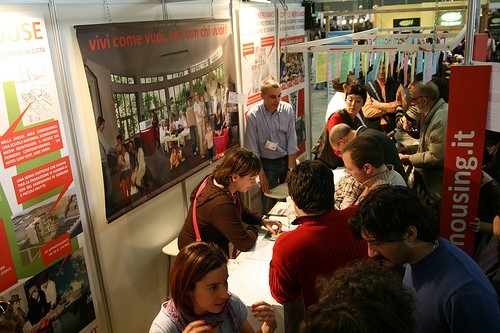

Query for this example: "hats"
[29,286,38,299]
[405,105,420,123]
[8,293,23,302]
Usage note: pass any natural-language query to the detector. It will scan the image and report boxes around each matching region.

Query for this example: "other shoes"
[201,152,206,158]
[209,159,212,164]
[194,146,198,155]
[146,190,151,198]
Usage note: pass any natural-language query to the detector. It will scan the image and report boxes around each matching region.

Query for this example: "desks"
[165,135,185,152]
[227,167,346,333]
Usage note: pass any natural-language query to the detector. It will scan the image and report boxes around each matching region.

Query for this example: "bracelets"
[287,167,294,172]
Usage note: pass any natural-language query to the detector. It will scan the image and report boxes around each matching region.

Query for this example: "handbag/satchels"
[107,153,115,167]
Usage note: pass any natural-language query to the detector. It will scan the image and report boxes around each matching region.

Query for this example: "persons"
[361,60,403,134]
[340,134,409,211]
[314,29,500,123]
[95,75,231,216]
[297,258,419,333]
[397,79,448,204]
[144,239,278,333]
[311,84,367,169]
[492,210,500,241]
[468,170,500,295]
[178,147,282,262]
[423,170,500,261]
[280,52,306,81]
[268,159,371,311]
[324,71,368,129]
[348,184,500,333]
[243,79,300,204]
[329,122,406,182]
[0,247,93,333]
[395,80,425,138]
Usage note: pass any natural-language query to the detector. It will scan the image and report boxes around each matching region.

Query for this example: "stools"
[162,238,180,296]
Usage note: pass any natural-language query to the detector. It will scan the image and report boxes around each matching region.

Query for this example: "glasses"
[410,96,428,103]
